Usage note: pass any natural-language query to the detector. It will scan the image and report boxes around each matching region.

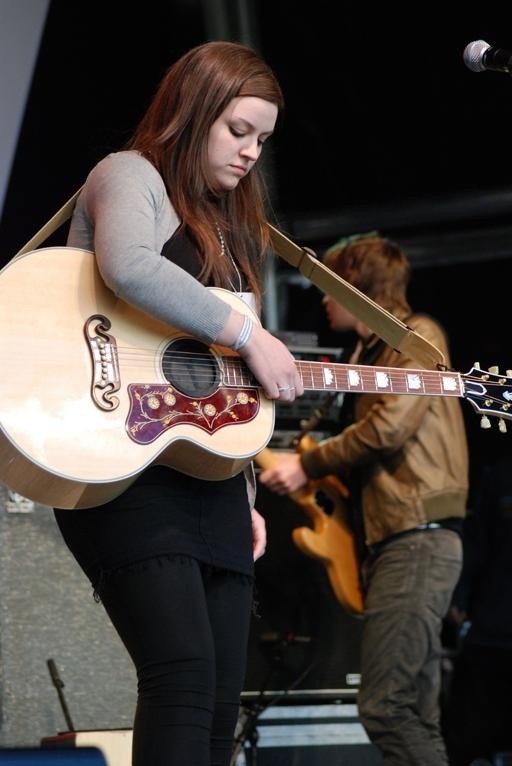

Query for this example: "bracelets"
[229,316,252,352]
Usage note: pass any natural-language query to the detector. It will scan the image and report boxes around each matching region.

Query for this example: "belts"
[366,515,467,558]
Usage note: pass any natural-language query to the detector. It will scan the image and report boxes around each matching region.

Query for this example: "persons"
[443,601,503,765]
[259,230,469,765]
[51,40,306,766]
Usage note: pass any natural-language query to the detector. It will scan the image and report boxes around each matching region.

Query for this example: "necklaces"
[213,221,225,257]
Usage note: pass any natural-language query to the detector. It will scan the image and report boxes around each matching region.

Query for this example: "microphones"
[463,39,512,73]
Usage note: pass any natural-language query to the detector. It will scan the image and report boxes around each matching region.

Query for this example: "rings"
[290,387,295,391]
[278,386,290,392]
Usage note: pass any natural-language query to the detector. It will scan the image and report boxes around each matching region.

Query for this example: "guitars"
[0,247,512,510]
[255,434,365,618]
